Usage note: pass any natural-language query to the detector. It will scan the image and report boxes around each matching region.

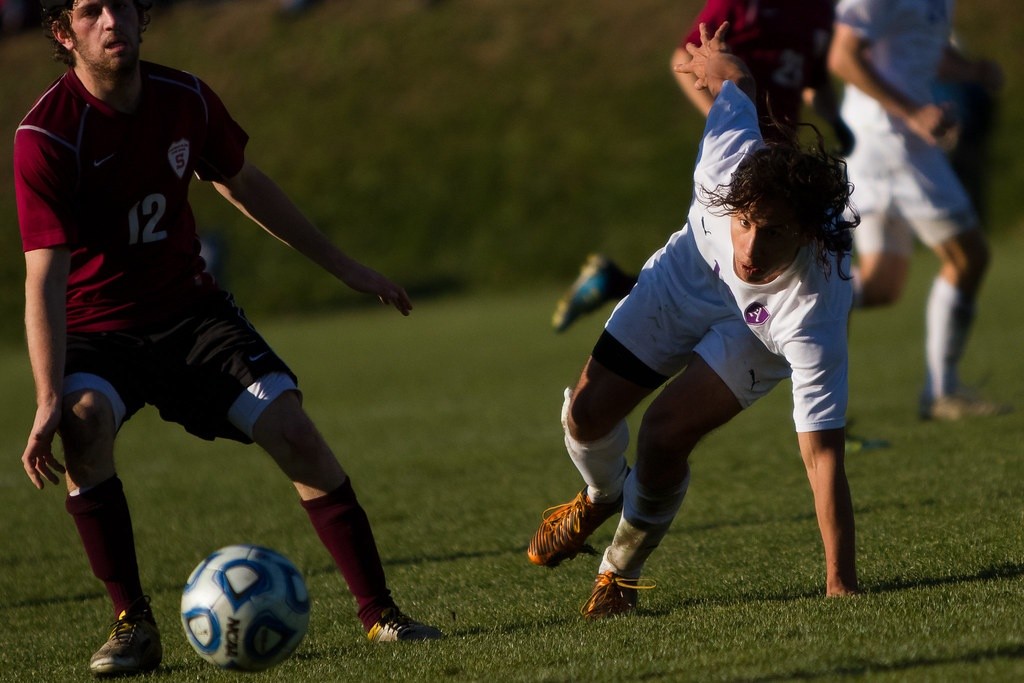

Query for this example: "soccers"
[178,542,310,674]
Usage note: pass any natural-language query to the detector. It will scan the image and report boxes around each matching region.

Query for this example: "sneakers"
[844,432,872,453]
[527,466,633,567]
[580,545,657,625]
[89,594,162,676]
[364,597,442,642]
[551,252,620,336]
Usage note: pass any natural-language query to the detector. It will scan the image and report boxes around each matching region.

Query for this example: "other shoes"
[926,396,1012,422]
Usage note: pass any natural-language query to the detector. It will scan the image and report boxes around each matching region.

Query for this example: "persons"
[11,0,439,679]
[523,0,987,616]
